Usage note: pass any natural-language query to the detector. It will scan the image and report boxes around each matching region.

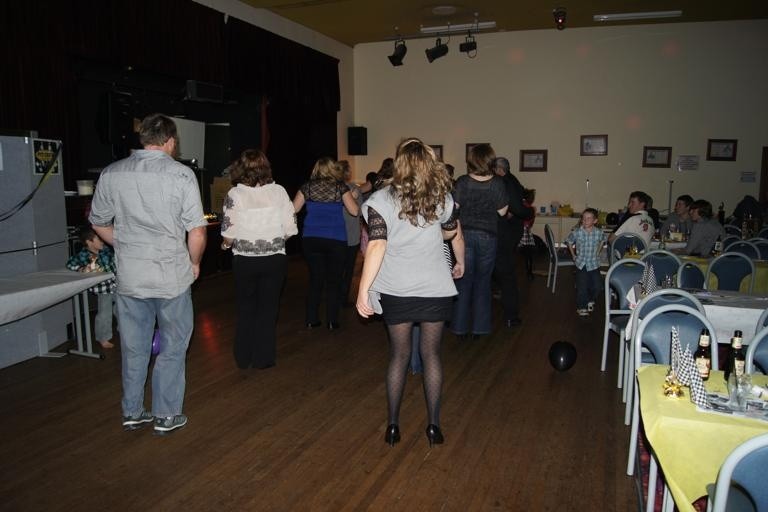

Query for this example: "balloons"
[597,212,625,226]
[548,341,578,371]
[151,329,163,354]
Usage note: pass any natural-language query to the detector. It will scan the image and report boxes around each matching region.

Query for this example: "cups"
[725,372,754,412]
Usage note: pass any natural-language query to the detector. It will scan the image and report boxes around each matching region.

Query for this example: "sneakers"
[153,415,188,432]
[122,411,155,426]
[576,308,589,317]
[587,301,595,312]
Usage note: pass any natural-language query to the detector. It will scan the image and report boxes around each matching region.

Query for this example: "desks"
[0,269,114,360]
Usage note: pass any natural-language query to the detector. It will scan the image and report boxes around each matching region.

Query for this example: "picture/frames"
[580,135,608,156]
[642,146,672,168]
[519,150,547,172]
[429,145,442,162]
[706,139,737,161]
[466,143,490,163]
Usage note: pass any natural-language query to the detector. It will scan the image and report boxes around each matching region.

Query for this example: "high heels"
[426,424,443,448]
[385,424,400,447]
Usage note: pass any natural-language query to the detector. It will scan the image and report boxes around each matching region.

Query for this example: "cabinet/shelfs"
[200,225,233,279]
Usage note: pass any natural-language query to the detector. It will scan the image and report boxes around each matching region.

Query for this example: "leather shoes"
[304,315,522,342]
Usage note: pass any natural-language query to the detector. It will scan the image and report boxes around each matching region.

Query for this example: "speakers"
[348,126,368,156]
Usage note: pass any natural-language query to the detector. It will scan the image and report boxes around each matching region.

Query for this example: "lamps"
[420,20,496,34]
[388,40,407,67]
[459,31,479,58]
[592,10,682,22]
[424,39,449,63]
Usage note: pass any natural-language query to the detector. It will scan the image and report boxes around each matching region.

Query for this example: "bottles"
[693,329,711,382]
[724,330,746,383]
[659,235,666,255]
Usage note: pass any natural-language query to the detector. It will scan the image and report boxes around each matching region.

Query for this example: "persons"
[442,139,538,339]
[563,208,608,317]
[408,218,466,373]
[355,137,465,449]
[88,113,208,435]
[336,150,395,309]
[220,147,299,370]
[672,201,726,257]
[294,157,359,331]
[64,232,120,349]
[661,195,695,241]
[607,192,661,265]
